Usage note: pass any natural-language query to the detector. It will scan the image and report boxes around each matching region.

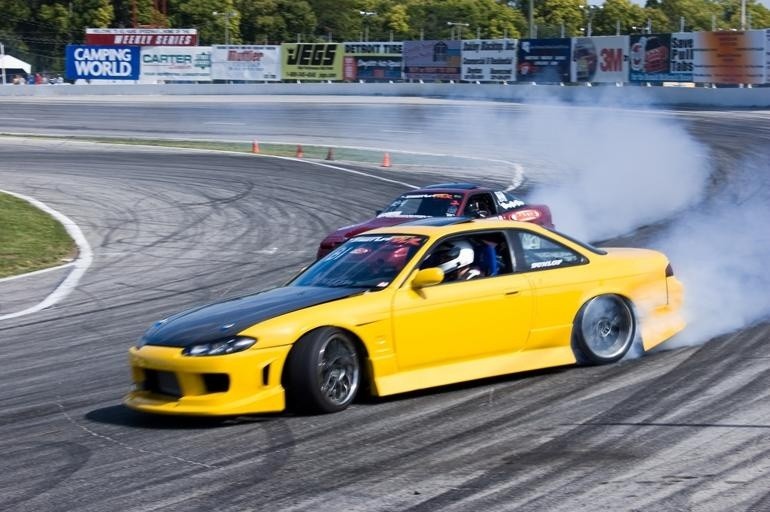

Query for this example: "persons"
[435,239,486,281]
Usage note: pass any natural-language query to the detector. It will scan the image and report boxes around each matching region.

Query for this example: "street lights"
[579,3,605,36]
[212,10,239,43]
[447,21,469,39]
[631,25,650,33]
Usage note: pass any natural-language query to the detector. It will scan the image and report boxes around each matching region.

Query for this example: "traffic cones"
[251,137,261,153]
[380,152,393,167]
[323,147,336,161]
[296,145,305,159]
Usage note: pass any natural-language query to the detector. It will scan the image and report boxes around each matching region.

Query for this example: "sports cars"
[122,220,686,421]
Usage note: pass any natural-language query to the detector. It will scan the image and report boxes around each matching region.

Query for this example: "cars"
[315,178,556,261]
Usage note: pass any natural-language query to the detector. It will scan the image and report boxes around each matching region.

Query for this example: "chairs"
[477,246,498,277]
